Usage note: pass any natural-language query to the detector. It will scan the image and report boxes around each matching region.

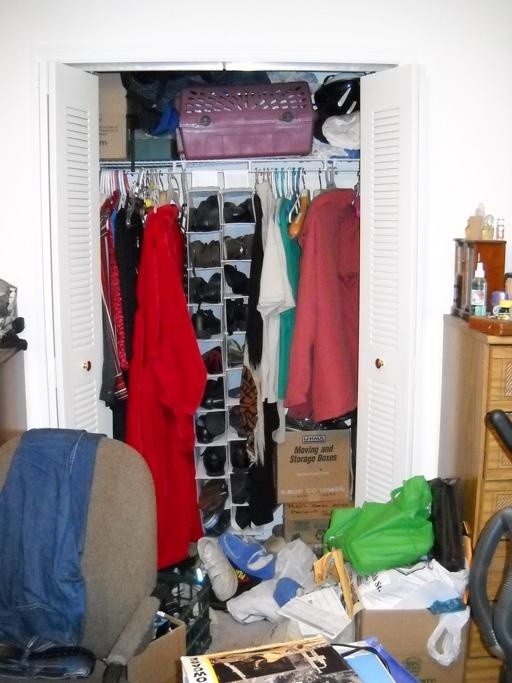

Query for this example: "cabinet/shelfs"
[441,315,512,681]
[1,344,24,444]
[39,57,420,508]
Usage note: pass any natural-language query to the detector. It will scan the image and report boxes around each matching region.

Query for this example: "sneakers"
[219,533,275,580]
[197,537,238,602]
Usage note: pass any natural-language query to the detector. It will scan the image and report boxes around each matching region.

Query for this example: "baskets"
[150,571,212,656]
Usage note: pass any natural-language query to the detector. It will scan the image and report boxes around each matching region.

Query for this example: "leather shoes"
[189,195,254,536]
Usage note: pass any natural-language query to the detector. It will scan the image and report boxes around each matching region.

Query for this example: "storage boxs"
[127,128,177,159]
[276,417,354,503]
[344,571,471,683]
[90,71,126,159]
[122,607,185,682]
[282,503,353,550]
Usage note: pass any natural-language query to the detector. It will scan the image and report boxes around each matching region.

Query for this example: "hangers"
[97,162,370,239]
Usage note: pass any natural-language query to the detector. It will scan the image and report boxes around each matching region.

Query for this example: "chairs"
[0,428,180,682]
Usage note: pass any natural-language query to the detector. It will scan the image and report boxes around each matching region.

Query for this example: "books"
[174,632,418,682]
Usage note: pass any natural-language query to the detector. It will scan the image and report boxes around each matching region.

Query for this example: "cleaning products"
[471,262,487,316]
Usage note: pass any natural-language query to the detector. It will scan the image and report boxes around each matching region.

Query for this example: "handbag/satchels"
[323,476,435,577]
[427,478,465,572]
